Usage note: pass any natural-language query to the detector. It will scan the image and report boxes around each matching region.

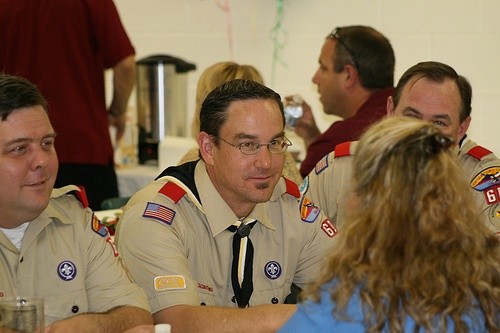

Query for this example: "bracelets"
[109,106,125,118]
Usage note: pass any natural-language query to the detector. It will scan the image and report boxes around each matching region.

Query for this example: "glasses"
[330,27,360,72]
[213,135,292,155]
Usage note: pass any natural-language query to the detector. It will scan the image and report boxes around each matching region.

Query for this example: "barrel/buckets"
[136,54,198,166]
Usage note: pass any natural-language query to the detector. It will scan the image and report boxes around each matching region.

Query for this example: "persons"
[285,24,398,181]
[274,115,500,333]
[300,61,500,238]
[112,78,344,333]
[0,75,155,333]
[182,61,303,188]
[0,0,138,210]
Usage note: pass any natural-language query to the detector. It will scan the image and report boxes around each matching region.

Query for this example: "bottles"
[284,94,305,132]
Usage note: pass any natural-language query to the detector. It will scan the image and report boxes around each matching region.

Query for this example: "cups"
[0,296,45,333]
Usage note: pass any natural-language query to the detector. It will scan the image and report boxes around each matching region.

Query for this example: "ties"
[153,160,254,308]
